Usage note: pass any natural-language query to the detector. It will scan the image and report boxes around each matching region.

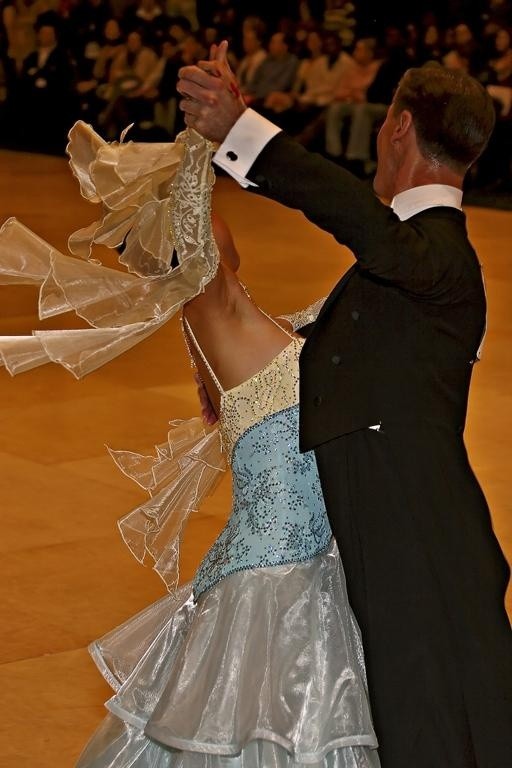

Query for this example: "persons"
[0,38,380,767]
[176,44,512,768]
[0,0,512,211]
